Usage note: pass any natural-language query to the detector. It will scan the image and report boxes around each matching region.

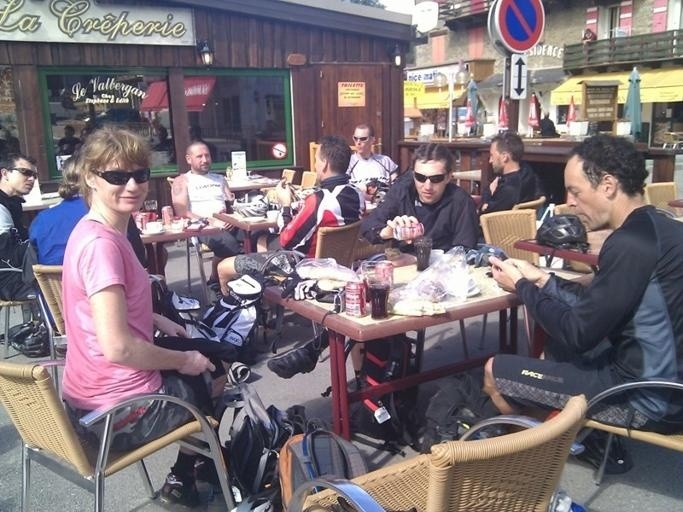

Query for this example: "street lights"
[433,68,472,142]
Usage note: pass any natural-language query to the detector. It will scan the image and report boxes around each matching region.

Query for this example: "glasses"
[413,171,448,183]
[6,167,37,179]
[352,136,372,142]
[89,167,150,185]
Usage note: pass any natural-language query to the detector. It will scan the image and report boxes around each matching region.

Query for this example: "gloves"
[465,246,508,268]
[280,271,317,300]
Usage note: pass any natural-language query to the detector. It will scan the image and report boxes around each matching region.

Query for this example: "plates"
[447,283,483,297]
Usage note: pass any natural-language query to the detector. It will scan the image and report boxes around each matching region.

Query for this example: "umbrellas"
[623,65,642,139]
[467,77,478,134]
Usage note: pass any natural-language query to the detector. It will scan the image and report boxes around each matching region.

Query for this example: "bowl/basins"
[140,217,189,233]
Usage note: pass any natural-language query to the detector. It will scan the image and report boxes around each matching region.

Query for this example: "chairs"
[2,169,682,512]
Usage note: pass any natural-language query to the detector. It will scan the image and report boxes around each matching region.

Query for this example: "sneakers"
[573,430,634,476]
[156,472,208,510]
[266,347,317,378]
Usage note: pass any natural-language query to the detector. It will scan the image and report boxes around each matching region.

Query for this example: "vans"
[454,107,472,134]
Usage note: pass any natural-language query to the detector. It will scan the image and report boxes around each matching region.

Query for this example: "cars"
[81,108,152,140]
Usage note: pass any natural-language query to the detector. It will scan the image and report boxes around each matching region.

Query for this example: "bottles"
[410,236,431,271]
[362,260,393,320]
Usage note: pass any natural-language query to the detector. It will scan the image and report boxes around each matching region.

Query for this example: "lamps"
[391,43,402,69]
[196,38,215,71]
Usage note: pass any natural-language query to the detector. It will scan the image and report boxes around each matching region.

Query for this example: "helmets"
[535,213,590,254]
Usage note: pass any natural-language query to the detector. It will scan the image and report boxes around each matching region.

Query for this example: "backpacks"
[197,383,313,512]
[320,333,421,457]
[277,418,368,512]
[200,273,263,347]
[151,274,220,351]
[0,320,50,358]
[407,372,484,453]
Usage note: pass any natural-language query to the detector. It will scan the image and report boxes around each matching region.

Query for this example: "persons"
[538,112,560,138]
[28,156,146,278]
[484,131,683,474]
[345,125,399,215]
[59,125,80,155]
[3,129,20,155]
[268,142,483,379]
[61,126,232,508]
[478,131,542,215]
[171,141,269,294]
[153,125,175,162]
[582,28,596,44]
[217,135,359,296]
[0,150,40,302]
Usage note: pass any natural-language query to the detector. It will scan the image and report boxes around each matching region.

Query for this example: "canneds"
[136,215,146,231]
[393,223,425,241]
[345,281,367,317]
[376,260,393,285]
[161,206,174,225]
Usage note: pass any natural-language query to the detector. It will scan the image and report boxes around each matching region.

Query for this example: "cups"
[143,199,158,214]
[428,248,444,262]
[267,210,280,221]
